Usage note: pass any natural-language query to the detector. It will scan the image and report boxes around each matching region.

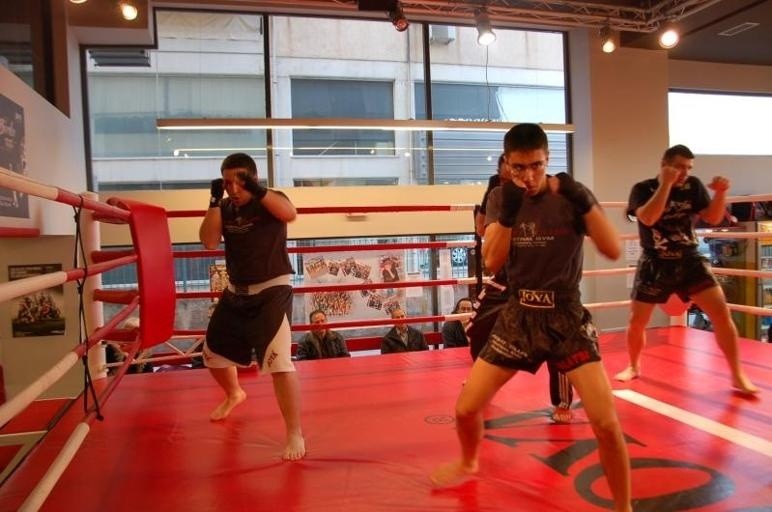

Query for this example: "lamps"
[597,13,683,55]
[382,2,413,34]
[469,8,497,50]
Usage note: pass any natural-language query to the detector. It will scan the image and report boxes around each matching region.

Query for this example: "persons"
[425,123,633,512]
[296,310,351,362]
[380,308,429,353]
[472,152,576,423]
[14,291,62,324]
[106,318,152,374]
[761,287,772,343]
[198,153,307,461]
[193,303,219,369]
[305,257,402,317]
[441,298,479,348]
[691,306,714,332]
[611,144,764,395]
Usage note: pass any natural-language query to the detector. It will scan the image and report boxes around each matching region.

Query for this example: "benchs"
[125,329,482,374]
